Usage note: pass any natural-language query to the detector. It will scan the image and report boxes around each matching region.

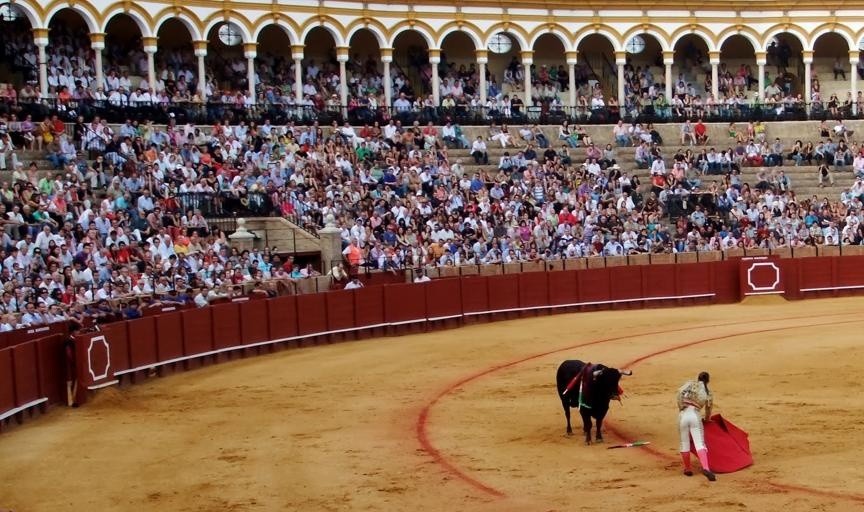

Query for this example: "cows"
[554,357,633,443]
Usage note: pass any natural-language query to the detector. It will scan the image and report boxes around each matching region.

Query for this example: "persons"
[0,33,864,334]
[676,372,716,482]
[61,324,81,407]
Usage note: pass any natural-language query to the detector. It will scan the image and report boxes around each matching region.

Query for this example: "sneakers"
[683,470,693,477]
[702,469,716,482]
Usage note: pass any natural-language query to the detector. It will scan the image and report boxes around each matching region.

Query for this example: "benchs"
[447,130,857,212]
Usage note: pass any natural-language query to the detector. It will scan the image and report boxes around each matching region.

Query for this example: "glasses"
[57,292,61,296]
[34,250,40,255]
[28,185,33,188]
[46,278,53,282]
[26,238,32,240]
[41,291,48,295]
